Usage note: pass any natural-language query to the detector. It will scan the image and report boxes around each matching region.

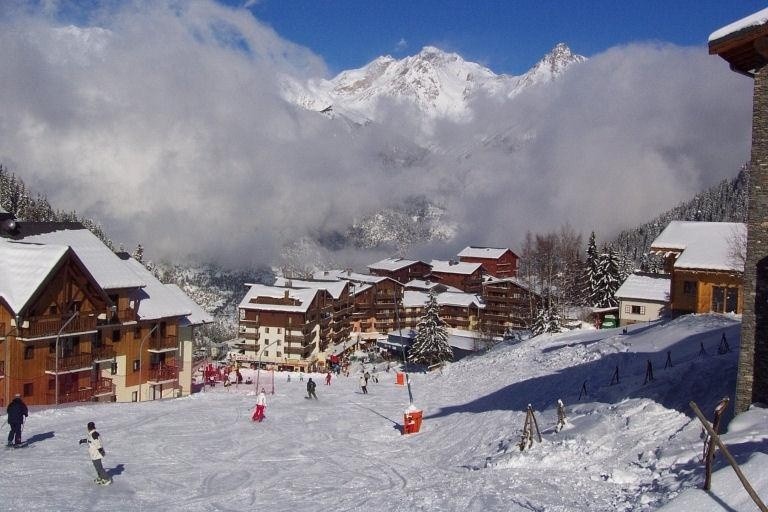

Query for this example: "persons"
[252,388,268,421]
[7,393,28,448]
[79,422,111,484]
[201,341,389,400]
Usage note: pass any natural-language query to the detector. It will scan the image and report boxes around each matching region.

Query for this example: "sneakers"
[101,479,112,486]
[95,477,101,483]
[8,442,22,447]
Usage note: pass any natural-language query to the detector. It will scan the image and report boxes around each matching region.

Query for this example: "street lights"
[55,311,79,405]
[139,324,158,401]
[256,339,279,395]
